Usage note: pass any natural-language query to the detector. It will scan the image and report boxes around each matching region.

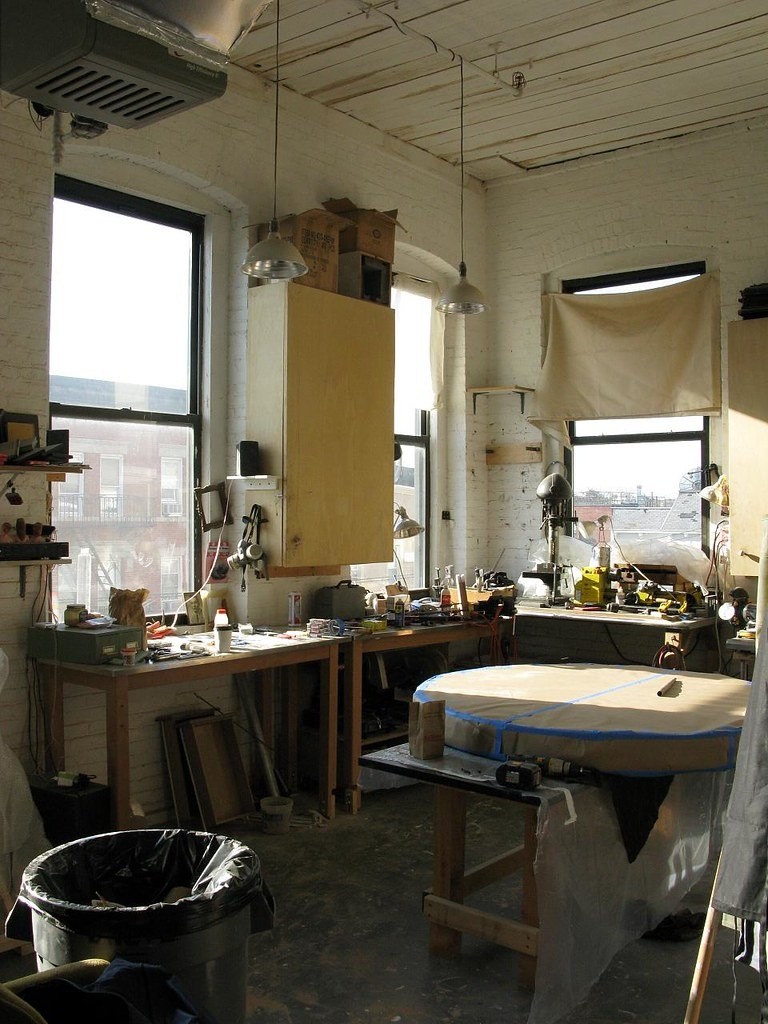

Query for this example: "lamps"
[239,0,310,283]
[430,50,491,322]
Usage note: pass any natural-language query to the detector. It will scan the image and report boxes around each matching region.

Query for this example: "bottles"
[395,599,405,628]
[213,608,228,637]
[64,603,86,626]
[440,584,451,612]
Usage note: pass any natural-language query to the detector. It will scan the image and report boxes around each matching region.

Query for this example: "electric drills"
[494,754,593,790]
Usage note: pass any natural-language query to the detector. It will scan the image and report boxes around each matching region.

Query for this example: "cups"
[121,647,137,665]
[215,624,232,652]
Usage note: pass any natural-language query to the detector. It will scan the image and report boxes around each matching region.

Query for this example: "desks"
[359,662,752,998]
[501,600,725,666]
[277,617,521,817]
[39,623,352,830]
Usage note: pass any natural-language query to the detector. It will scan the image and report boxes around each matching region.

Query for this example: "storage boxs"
[338,197,409,261]
[260,206,347,293]
[33,774,112,830]
[21,617,147,666]
[0,541,69,560]
[450,584,516,605]
[335,250,395,308]
[298,727,410,796]
[288,591,302,627]
[169,711,259,831]
[152,706,223,825]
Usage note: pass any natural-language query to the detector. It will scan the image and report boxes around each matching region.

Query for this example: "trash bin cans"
[5,826,276,1024]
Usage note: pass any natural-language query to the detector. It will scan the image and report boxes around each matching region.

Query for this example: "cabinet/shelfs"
[0,466,91,568]
[244,281,397,571]
[721,318,768,581]
